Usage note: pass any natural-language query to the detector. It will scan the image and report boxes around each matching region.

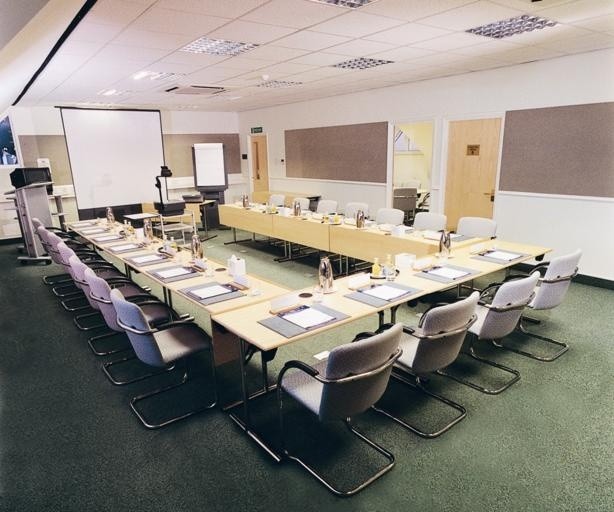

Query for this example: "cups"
[328,213,345,225]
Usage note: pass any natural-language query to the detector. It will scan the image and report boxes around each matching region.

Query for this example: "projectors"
[183,194,206,202]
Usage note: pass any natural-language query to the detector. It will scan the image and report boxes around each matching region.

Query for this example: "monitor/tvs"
[10,167,52,189]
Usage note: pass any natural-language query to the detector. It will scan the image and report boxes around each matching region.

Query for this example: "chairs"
[280,324,405,494]
[489,249,582,362]
[31,190,552,463]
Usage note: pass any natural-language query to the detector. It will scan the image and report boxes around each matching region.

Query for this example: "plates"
[240,206,256,209]
[189,259,207,264]
[291,215,304,219]
[355,227,369,230]
[315,288,339,294]
[104,224,119,227]
[433,252,455,258]
[141,238,156,242]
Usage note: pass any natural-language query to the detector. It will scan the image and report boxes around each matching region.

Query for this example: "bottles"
[293,199,301,218]
[356,208,365,229]
[191,234,203,261]
[385,255,395,282]
[105,207,115,225]
[161,235,177,253]
[371,258,381,276]
[265,201,278,214]
[122,219,134,234]
[143,219,154,241]
[318,253,333,289]
[242,194,249,208]
[438,230,453,256]
